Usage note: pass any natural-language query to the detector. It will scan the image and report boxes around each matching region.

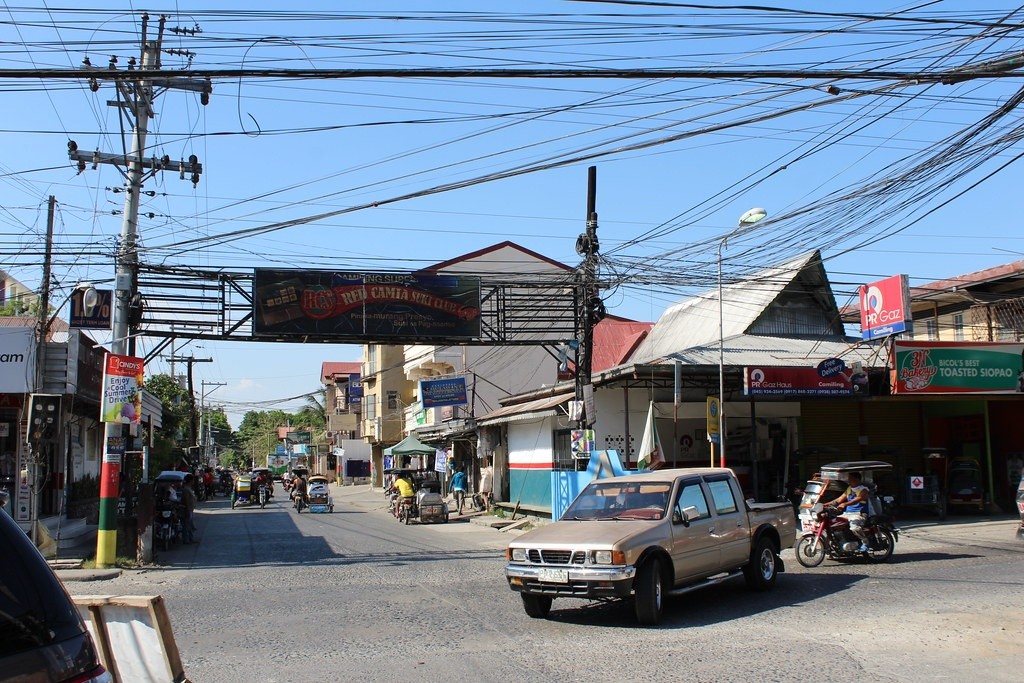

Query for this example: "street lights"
[28,281,95,410]
[716,209,772,467]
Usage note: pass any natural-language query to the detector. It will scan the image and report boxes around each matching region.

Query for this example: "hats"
[457,468,463,472]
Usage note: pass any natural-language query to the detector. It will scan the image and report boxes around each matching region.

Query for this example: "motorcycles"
[795,501,899,570]
[798,461,898,530]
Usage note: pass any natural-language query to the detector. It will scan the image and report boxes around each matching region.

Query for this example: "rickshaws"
[236,467,278,509]
[152,471,199,552]
[392,476,453,525]
[287,466,335,515]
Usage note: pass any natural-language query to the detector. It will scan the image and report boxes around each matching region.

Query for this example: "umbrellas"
[637,401,665,470]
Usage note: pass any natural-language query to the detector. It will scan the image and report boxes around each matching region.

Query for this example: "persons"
[448,468,467,515]
[191,462,306,506]
[389,473,414,518]
[1007,454,1024,516]
[117,471,201,544]
[822,472,880,552]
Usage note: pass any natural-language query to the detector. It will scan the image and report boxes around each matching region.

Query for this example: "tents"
[384,434,436,455]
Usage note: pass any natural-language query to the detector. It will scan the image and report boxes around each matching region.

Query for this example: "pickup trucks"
[502,469,801,626]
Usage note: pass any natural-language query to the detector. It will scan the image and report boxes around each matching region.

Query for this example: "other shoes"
[458,513,463,515]
[183,542,192,545]
[190,539,200,543]
[293,503,296,507]
[305,503,309,507]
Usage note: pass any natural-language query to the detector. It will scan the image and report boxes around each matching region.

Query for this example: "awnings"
[477,393,576,427]
